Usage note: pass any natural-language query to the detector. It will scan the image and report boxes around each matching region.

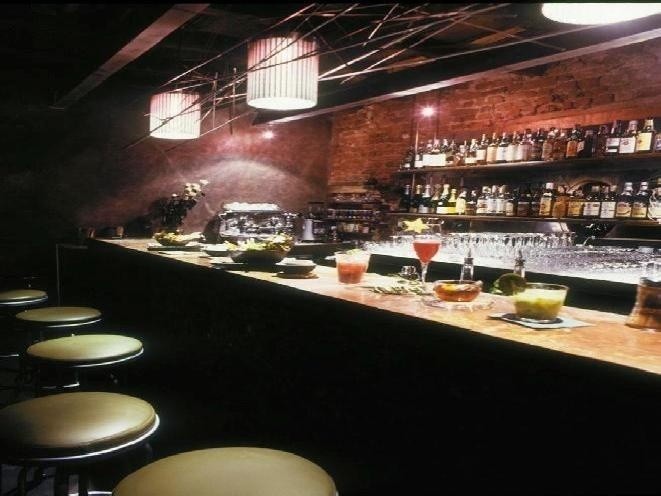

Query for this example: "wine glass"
[388,231,574,267]
[412,222,441,292]
[523,243,660,282]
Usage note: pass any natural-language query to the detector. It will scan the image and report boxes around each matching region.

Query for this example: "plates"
[431,278,484,302]
[202,250,228,257]
[209,262,244,268]
[156,239,190,245]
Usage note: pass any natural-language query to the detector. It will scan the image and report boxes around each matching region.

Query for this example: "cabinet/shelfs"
[387,152,661,226]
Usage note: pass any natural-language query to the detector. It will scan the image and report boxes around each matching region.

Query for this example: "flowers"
[160,179,211,226]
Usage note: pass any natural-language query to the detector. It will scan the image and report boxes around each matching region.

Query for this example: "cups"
[332,248,370,288]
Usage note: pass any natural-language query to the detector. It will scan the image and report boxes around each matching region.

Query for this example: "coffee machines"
[297,200,329,243]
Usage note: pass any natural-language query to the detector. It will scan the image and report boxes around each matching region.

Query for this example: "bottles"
[511,255,526,280]
[402,116,661,169]
[459,257,475,282]
[398,176,661,220]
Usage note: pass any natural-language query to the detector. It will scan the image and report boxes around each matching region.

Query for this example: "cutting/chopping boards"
[147,242,202,251]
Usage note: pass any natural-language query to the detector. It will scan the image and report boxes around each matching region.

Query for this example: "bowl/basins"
[509,280,569,323]
[274,262,316,276]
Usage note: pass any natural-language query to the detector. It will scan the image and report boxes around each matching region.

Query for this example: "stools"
[0,289,49,370]
[15,306,105,388]
[17,333,144,397]
[0,391,160,496]
[112,447,340,496]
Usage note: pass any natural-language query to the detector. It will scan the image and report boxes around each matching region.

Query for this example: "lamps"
[247,34,320,110]
[149,92,202,140]
